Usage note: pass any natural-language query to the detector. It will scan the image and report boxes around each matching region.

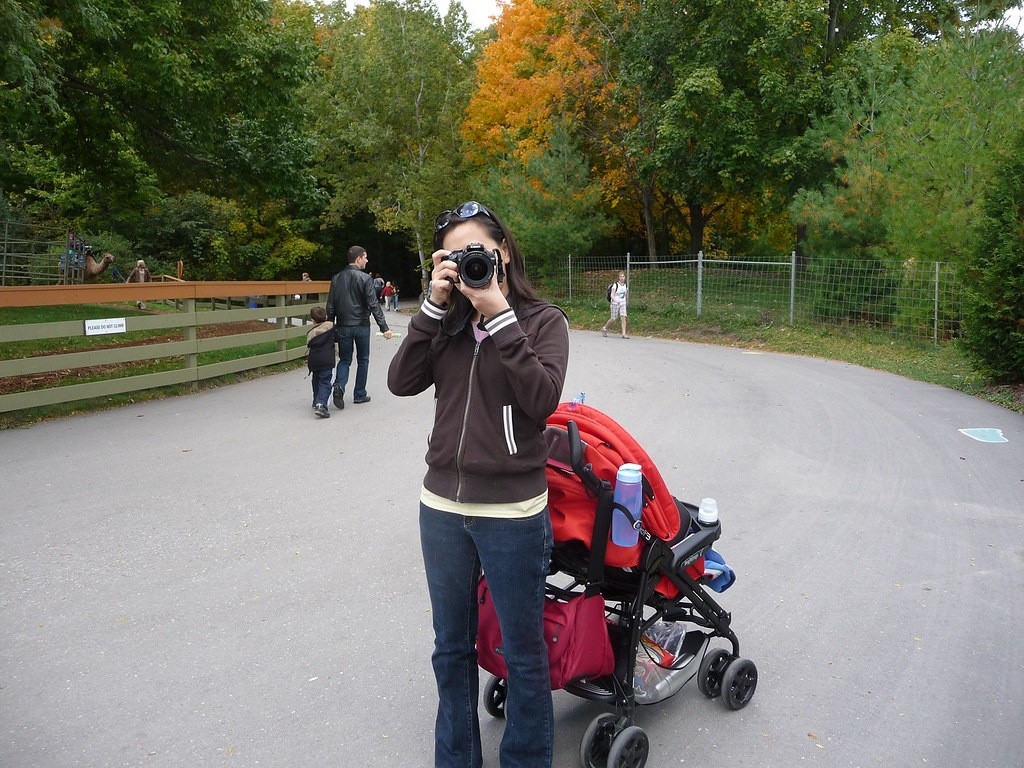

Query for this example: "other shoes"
[394,308,398,311]
[333,385,344,409]
[387,308,390,311]
[622,334,629,339]
[601,327,607,337]
[354,396,371,403]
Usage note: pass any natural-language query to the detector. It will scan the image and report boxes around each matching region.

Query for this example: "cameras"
[441,243,497,287]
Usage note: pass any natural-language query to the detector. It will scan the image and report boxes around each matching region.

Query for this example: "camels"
[56,251,116,285]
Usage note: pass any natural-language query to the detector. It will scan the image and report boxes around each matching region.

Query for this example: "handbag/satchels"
[475,574,615,691]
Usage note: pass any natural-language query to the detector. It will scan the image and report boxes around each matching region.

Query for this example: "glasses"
[434,201,490,244]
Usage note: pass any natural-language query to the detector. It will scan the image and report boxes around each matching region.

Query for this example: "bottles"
[698,497,718,526]
[612,462,642,546]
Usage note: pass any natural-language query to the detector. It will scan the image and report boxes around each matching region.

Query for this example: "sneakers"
[315,403,330,418]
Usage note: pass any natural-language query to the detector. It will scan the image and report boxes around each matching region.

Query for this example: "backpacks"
[607,281,627,302]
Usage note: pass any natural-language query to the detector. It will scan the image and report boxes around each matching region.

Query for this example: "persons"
[602,270,629,339]
[126,260,152,309]
[302,272,399,312]
[387,199,571,768]
[326,245,392,410]
[306,306,339,417]
